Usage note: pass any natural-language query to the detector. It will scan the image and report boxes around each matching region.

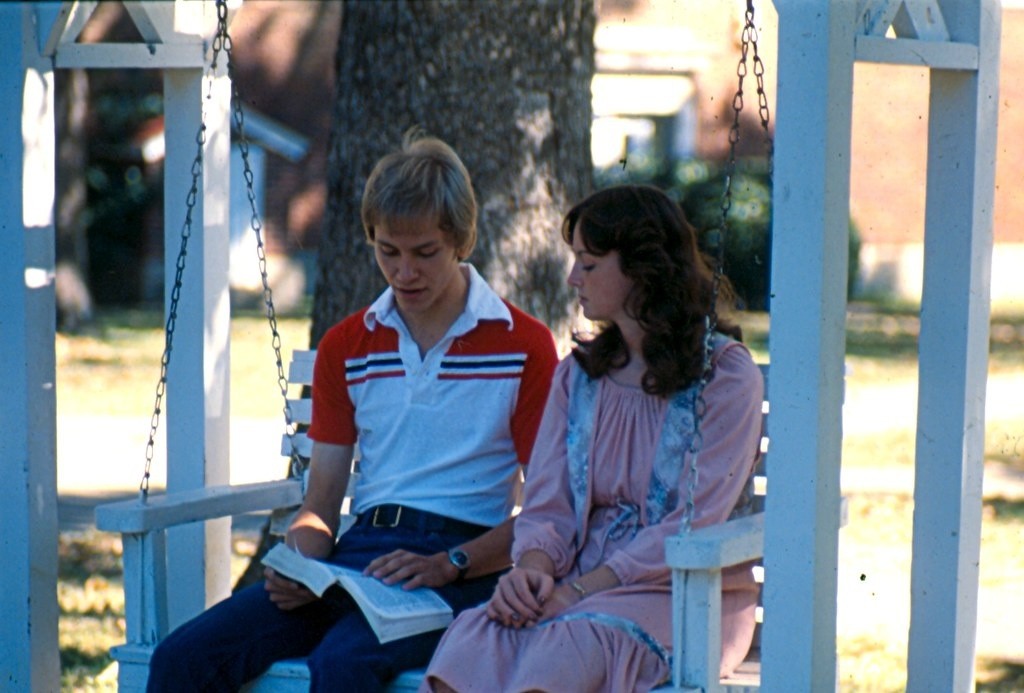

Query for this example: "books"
[260,539,454,644]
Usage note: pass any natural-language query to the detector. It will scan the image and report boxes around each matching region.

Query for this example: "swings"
[93,0,773,693]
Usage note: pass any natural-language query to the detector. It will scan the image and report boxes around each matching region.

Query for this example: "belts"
[356,503,492,536]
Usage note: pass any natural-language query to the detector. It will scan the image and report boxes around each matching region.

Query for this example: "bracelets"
[569,581,587,596]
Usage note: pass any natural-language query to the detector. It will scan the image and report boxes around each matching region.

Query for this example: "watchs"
[447,547,470,579]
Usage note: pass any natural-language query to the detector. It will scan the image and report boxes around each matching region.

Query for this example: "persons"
[416,188,765,693]
[143,141,563,692]
